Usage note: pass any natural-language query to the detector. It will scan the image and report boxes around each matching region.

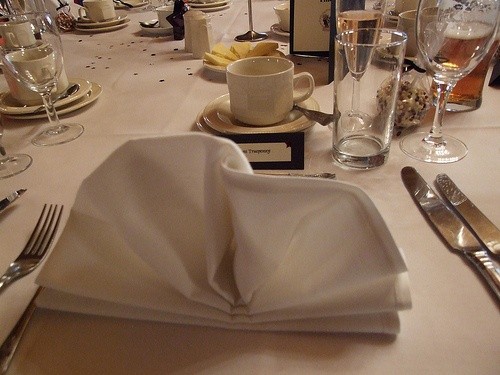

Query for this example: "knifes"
[436,172,500,255]
[401,165,500,296]
[0,188,28,211]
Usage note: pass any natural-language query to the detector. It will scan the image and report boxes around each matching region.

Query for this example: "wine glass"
[0,115,33,180]
[0,12,85,147]
[234,0,268,42]
[400,0,500,164]
[328,0,387,131]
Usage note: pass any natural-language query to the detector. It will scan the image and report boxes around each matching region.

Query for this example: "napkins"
[33,133,414,334]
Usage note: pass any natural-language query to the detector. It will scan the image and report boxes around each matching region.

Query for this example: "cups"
[429,24,500,112]
[78,0,116,22]
[156,6,174,27]
[397,10,419,57]
[1,22,36,48]
[225,57,315,125]
[0,47,68,106]
[331,28,407,169]
[272,3,290,32]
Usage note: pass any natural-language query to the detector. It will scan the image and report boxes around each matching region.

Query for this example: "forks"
[0,204,63,290]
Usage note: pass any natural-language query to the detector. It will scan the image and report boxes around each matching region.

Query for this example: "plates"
[75,12,132,32]
[0,79,103,119]
[187,0,232,12]
[195,92,320,136]
[271,23,290,36]
[140,22,173,35]
[202,47,286,74]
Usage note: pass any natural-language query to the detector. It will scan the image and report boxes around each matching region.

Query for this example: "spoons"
[35,84,79,114]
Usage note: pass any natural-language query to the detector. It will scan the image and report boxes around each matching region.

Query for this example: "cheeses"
[203,42,279,66]
